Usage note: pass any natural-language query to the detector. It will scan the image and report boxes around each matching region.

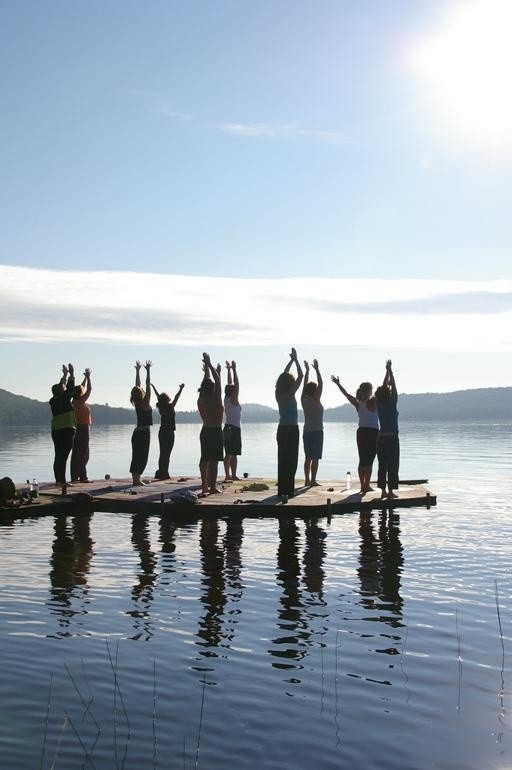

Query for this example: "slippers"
[6,497,41,506]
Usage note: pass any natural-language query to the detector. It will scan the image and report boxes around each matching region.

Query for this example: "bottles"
[346,471,351,490]
[23,477,40,504]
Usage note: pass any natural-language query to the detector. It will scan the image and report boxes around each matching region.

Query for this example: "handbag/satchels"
[170,490,198,505]
[0,477,15,506]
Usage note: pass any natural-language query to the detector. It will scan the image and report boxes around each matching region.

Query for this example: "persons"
[128,359,154,486]
[196,352,242,497]
[374,360,400,500]
[330,374,391,492]
[49,363,75,488]
[301,360,324,487]
[149,382,186,480]
[274,348,304,499]
[70,367,92,484]
